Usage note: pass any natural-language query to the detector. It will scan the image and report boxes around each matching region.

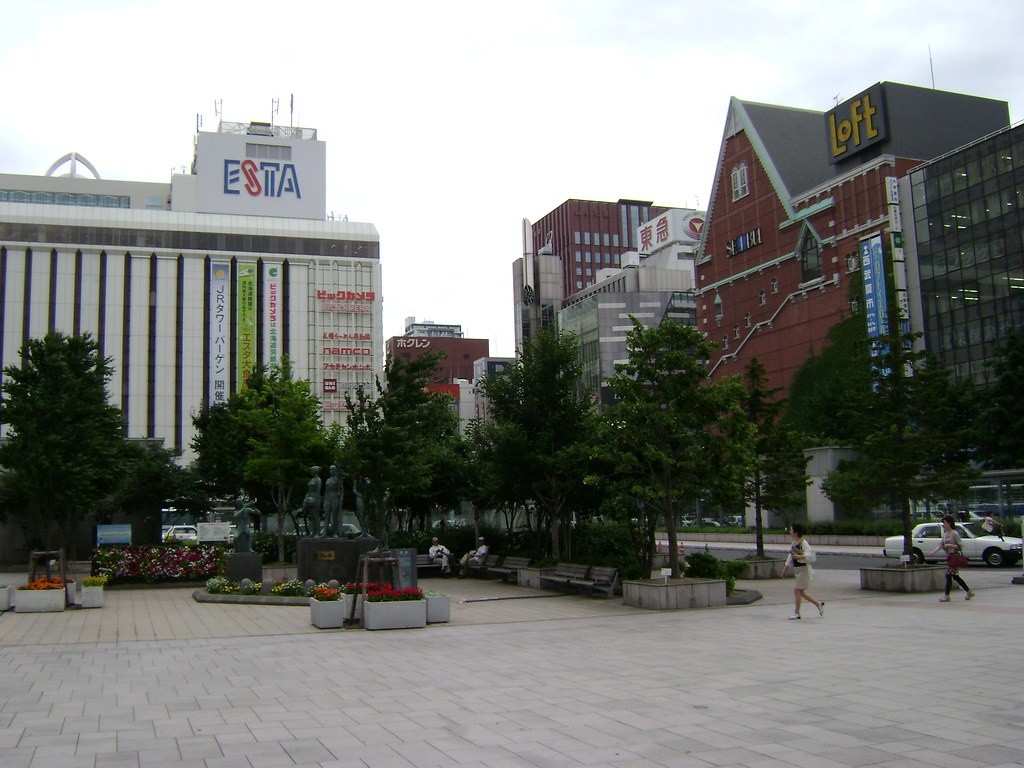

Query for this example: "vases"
[363,599,426,630]
[81,585,103,608]
[15,587,66,612]
[310,597,343,628]
[67,582,76,604]
[340,593,368,619]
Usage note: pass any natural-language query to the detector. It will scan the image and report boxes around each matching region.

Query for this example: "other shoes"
[939,597,950,602]
[965,591,974,600]
[457,576,464,579]
[819,601,824,616]
[789,613,801,620]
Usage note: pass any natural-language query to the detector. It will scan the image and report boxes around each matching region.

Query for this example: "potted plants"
[424,589,451,622]
[0,585,11,611]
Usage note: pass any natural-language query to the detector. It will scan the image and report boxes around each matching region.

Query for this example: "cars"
[950,511,992,522]
[883,522,1023,567]
[433,520,470,528]
[285,523,361,537]
[162,524,198,541]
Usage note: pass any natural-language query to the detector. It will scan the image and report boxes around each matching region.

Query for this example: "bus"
[161,509,210,540]
[936,484,1024,515]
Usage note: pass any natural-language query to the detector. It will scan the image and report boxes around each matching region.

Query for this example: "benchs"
[469,554,500,579]
[537,562,590,595]
[417,553,455,579]
[570,566,619,599]
[487,556,532,585]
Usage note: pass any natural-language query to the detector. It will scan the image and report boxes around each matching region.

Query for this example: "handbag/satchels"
[982,518,993,533]
[947,544,969,568]
[797,538,806,563]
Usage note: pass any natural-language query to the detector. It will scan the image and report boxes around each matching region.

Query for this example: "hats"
[432,537,438,542]
[478,537,485,541]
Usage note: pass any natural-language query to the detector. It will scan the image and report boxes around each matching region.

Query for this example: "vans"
[910,510,945,522]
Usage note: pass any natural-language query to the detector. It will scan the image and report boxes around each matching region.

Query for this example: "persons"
[353,475,371,537]
[319,465,344,538]
[184,528,189,533]
[928,515,975,601]
[428,537,451,579]
[780,523,825,620]
[459,537,487,575]
[981,510,1004,535]
[302,466,321,538]
[233,498,261,553]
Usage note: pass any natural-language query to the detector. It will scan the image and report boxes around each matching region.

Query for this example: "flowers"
[313,586,339,601]
[341,582,380,594]
[81,576,108,587]
[16,576,74,590]
[365,583,423,602]
